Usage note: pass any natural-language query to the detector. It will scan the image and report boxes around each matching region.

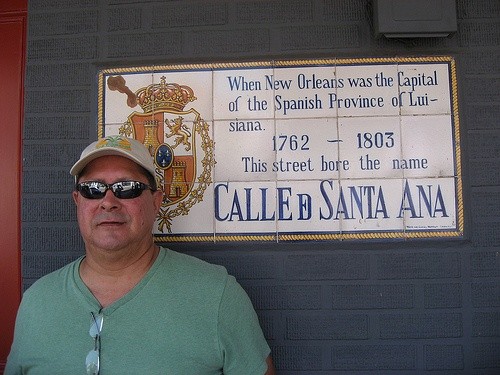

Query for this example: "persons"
[4,136,275,374]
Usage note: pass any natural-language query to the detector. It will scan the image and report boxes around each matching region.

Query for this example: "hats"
[69,136,157,189]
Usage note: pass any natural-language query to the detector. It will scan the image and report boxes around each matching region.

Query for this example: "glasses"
[74,181,149,200]
[86,310,104,374]
